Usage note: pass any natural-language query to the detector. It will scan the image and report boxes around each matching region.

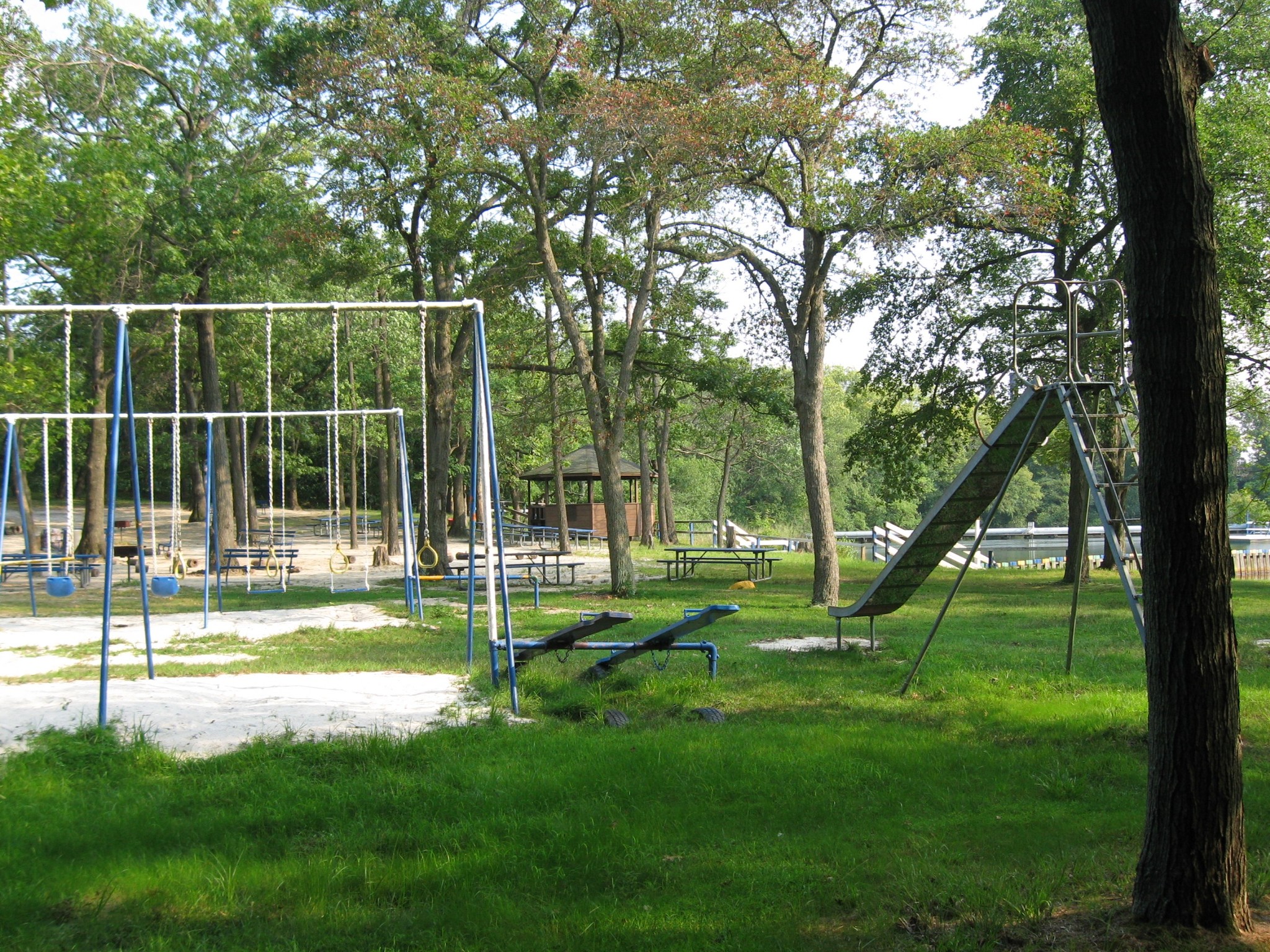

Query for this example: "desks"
[456,552,572,586]
[664,548,777,583]
[2,554,101,587]
[311,516,371,536]
[476,522,597,548]
[368,518,419,538]
[114,546,138,581]
[114,521,131,540]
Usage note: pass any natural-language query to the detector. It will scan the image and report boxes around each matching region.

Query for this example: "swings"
[149,416,178,596]
[327,414,371,594]
[43,418,74,597]
[241,415,289,597]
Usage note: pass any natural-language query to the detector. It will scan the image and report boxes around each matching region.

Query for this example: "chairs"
[158,539,171,551]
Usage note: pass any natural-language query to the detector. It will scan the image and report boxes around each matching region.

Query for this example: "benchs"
[2,563,101,588]
[446,563,585,591]
[656,557,783,582]
[465,528,607,549]
[307,520,418,539]
[220,530,299,585]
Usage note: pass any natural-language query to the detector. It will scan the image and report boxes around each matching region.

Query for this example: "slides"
[829,384,1105,617]
